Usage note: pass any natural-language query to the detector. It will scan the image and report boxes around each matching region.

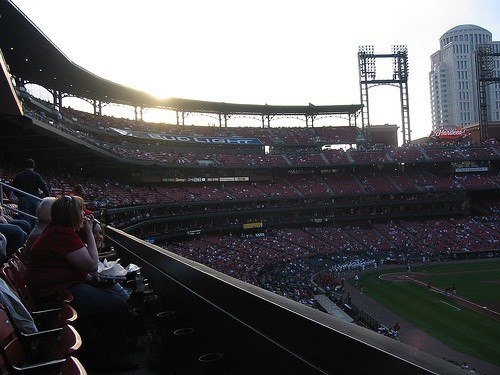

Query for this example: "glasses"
[64,194,72,207]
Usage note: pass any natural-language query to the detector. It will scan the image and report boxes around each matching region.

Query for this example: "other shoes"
[112,361,139,370]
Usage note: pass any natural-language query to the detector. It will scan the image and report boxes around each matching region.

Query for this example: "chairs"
[0,248,83,375]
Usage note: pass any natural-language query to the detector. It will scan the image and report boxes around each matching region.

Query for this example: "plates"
[96,273,126,279]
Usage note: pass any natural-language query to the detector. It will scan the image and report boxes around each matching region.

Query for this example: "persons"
[38,164,500,307]
[24,184,103,318]
[14,97,500,165]
[377,322,401,339]
[0,159,49,263]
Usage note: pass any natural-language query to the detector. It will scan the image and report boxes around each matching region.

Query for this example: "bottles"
[111,280,132,304]
[134,269,146,291]
[142,278,155,302]
